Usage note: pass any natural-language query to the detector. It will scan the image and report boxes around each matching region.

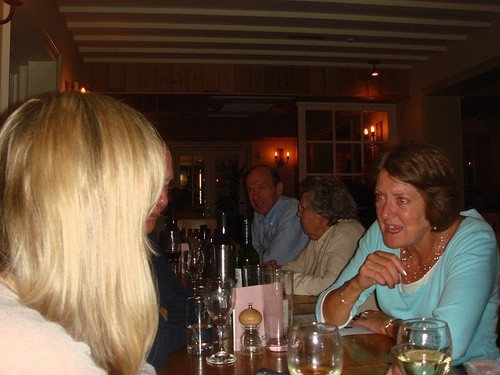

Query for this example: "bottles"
[212,211,235,314]
[234,217,260,286]
[160,213,216,279]
[240,326,263,356]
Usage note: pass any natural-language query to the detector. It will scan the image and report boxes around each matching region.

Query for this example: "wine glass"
[182,250,205,299]
[203,278,238,365]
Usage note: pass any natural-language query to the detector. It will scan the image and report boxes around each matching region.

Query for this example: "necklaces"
[401,231,446,283]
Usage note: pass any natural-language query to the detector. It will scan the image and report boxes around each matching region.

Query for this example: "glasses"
[297,204,313,212]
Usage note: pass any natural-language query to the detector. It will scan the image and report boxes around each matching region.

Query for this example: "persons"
[246,164,311,269]
[315,144,500,367]
[0,91,168,375]
[264,177,379,317]
[141,148,212,375]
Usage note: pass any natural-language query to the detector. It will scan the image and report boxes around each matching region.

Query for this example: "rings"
[363,314,366,317]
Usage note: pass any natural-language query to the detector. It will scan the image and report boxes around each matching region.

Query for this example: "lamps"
[363,122,384,148]
[274,148,289,168]
[367,60,382,77]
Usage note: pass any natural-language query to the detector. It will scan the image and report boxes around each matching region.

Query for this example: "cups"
[244,265,273,286]
[185,297,214,355]
[287,323,344,375]
[166,230,181,261]
[262,269,295,352]
[391,343,434,375]
[395,318,453,375]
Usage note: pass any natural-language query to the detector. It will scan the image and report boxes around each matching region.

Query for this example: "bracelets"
[340,280,349,303]
[384,318,402,333]
